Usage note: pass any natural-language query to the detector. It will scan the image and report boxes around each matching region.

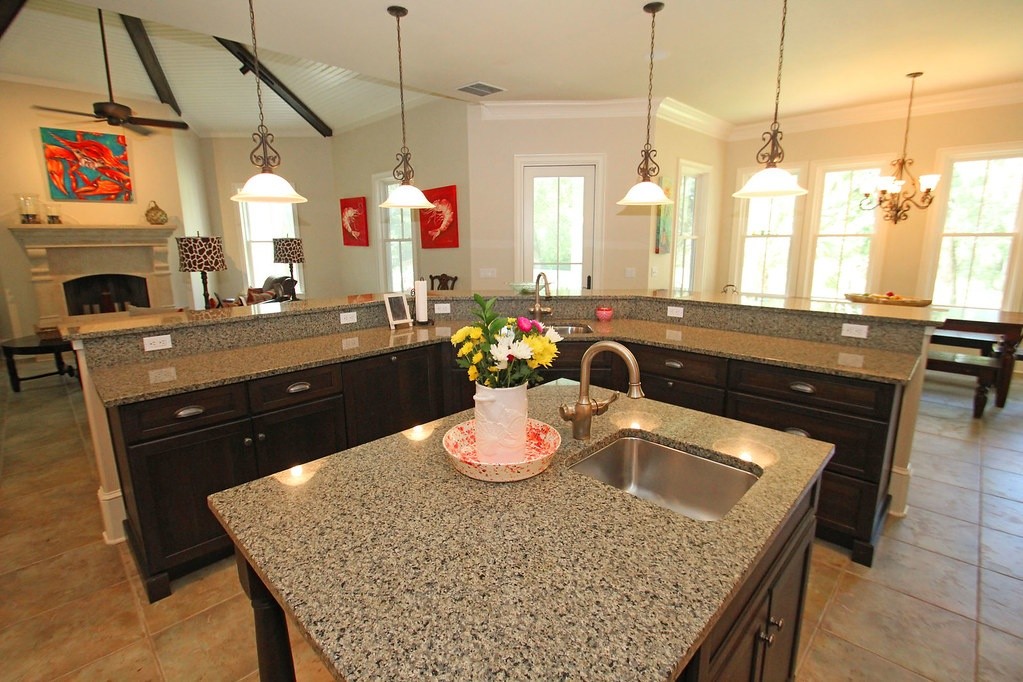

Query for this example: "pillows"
[246,289,275,302]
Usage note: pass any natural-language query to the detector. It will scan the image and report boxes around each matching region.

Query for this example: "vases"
[468,387,530,465]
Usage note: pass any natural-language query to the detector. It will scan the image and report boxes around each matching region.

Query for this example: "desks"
[924,304,1022,407]
[0,328,93,393]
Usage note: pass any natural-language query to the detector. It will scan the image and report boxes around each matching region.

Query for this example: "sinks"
[548,325,588,333]
[569,437,759,522]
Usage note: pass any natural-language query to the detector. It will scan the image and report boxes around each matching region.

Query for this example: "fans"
[29,15,189,137]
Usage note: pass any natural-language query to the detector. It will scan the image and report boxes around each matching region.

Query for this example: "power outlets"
[340,312,358,324]
[666,306,685,318]
[142,334,173,352]
[435,326,451,337]
[342,337,359,350]
[840,323,870,339]
[434,303,451,314]
[149,366,176,384]
[837,352,865,368]
[666,329,683,341]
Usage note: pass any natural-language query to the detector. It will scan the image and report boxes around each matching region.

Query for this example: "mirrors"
[383,291,414,331]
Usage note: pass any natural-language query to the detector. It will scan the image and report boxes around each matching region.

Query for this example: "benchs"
[925,348,1001,420]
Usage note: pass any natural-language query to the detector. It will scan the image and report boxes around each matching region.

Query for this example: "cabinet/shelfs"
[100,363,344,604]
[445,341,613,414]
[723,359,903,568]
[343,339,447,457]
[615,339,723,429]
[696,479,818,682]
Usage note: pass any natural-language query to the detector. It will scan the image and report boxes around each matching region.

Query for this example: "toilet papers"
[414,280,427,322]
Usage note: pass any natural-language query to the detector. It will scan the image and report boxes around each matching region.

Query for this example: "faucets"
[529,272,553,322]
[559,340,645,440]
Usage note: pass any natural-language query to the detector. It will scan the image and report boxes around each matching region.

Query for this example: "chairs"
[429,272,457,292]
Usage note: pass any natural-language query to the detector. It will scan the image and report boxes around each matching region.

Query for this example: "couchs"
[238,276,297,306]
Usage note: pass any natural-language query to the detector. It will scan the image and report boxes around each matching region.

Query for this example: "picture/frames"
[32,122,136,203]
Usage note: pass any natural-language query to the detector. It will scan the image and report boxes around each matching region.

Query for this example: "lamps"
[852,72,942,228]
[616,3,674,208]
[378,4,436,212]
[231,0,309,205]
[272,234,306,303]
[175,229,228,313]
[732,0,810,200]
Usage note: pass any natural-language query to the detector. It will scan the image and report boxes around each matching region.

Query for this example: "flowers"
[451,297,564,386]
